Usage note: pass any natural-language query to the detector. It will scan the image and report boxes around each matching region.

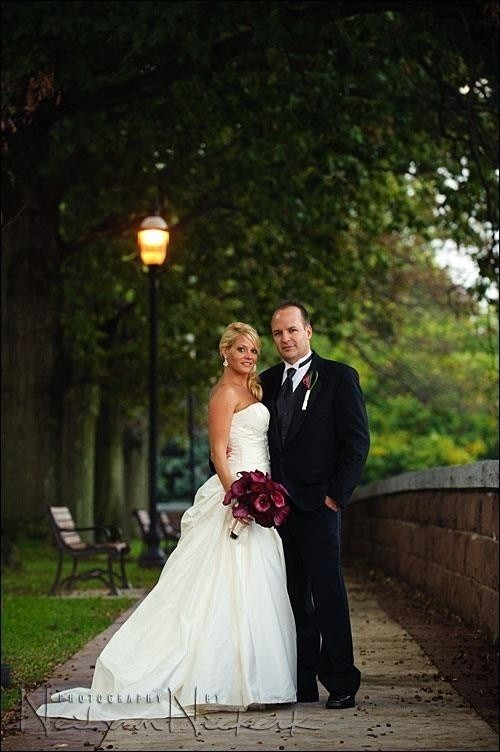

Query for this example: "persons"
[208,299,371,710]
[204,321,296,713]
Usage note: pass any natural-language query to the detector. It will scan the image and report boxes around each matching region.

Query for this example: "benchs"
[134,506,185,563]
[45,504,132,599]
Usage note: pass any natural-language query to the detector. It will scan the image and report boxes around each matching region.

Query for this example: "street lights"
[185,334,200,504]
[136,215,170,568]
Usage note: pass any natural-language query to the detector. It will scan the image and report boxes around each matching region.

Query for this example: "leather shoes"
[297,693,319,702]
[326,691,356,708]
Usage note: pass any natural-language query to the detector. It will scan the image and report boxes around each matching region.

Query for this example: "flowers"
[221,468,294,540]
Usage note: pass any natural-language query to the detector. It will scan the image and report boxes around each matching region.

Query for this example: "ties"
[283,354,313,396]
